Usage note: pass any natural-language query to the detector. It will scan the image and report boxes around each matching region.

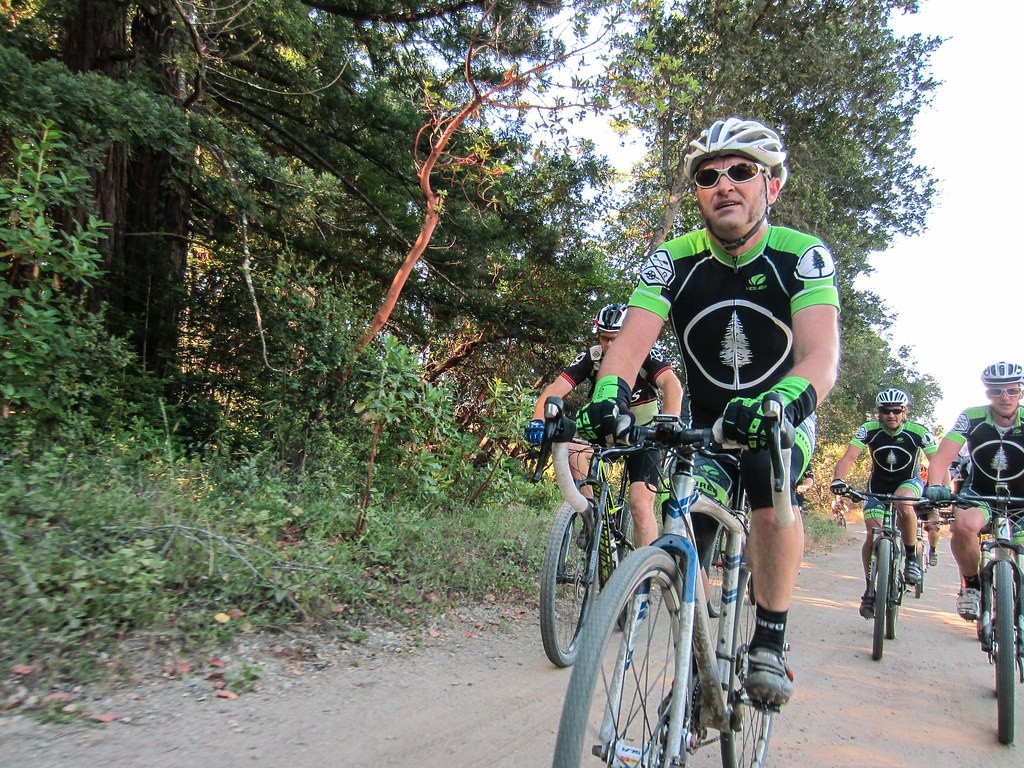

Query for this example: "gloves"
[926,484,953,508]
[653,420,688,448]
[524,419,544,444]
[576,375,634,447]
[712,376,817,453]
[830,479,853,496]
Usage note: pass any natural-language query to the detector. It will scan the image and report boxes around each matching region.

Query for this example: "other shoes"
[577,513,598,547]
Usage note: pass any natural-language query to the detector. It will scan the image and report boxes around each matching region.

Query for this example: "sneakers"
[905,556,923,583]
[928,550,938,566]
[742,646,794,709]
[860,587,875,618]
[657,672,702,727]
[957,588,982,621]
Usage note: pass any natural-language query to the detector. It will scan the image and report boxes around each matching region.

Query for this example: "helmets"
[875,388,909,408]
[594,302,629,333]
[981,361,1024,385]
[684,115,788,193]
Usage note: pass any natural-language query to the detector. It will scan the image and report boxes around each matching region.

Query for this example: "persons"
[522,303,682,619]
[576,118,840,726]
[830,360,1024,619]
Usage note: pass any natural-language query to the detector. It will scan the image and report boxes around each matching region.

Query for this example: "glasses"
[879,408,905,415]
[692,162,771,189]
[988,386,1024,397]
[598,336,617,343]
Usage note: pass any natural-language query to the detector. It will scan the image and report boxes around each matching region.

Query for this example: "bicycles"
[531,393,791,768]
[844,484,1024,745]
[834,508,848,527]
